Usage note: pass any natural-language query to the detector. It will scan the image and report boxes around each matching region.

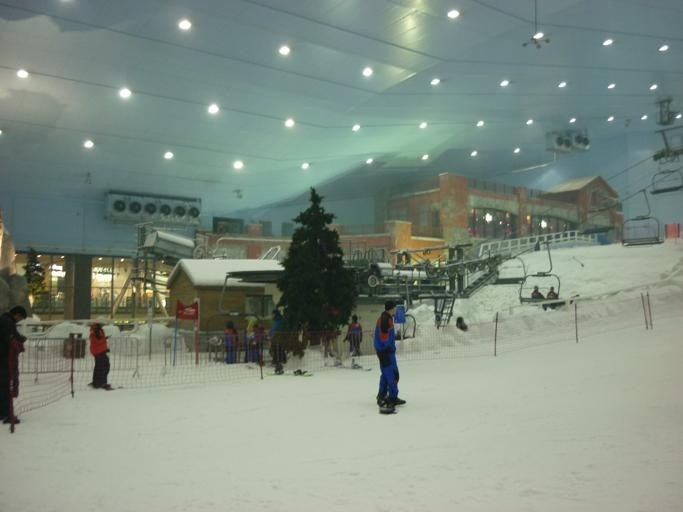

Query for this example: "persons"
[222,308,311,376]
[532,286,547,310]
[342,314,362,356]
[547,287,558,309]
[374,300,406,405]
[0,304,28,424]
[89,319,113,388]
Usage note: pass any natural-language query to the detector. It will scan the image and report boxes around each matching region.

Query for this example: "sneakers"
[93,382,111,389]
[377,394,406,408]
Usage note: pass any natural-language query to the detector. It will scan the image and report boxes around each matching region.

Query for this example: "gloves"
[379,351,391,366]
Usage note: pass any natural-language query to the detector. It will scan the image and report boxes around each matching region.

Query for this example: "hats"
[385,300,396,310]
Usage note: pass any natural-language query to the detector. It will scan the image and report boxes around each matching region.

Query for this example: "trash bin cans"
[63,332,86,359]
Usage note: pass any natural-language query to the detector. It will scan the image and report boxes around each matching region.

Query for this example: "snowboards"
[338,363,372,371]
[269,370,312,376]
[379,407,395,414]
